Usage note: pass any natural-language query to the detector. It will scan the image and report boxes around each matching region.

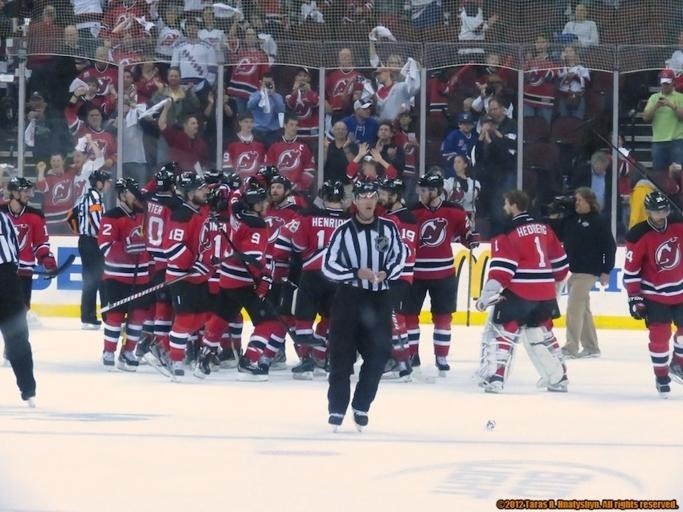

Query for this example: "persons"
[0,161,45,408]
[623,190,683,394]
[0,0,683,382]
[475,190,574,393]
[322,180,407,427]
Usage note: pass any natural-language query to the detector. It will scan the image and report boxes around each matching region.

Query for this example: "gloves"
[630,298,646,322]
[466,231,480,248]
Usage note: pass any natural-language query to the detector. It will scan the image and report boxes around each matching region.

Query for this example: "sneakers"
[561,346,576,356]
[327,413,346,426]
[577,349,600,358]
[353,408,369,425]
[489,374,504,387]
[672,353,683,380]
[656,373,670,394]
[558,374,568,385]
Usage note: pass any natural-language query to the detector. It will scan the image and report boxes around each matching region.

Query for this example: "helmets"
[7,175,37,192]
[645,192,670,213]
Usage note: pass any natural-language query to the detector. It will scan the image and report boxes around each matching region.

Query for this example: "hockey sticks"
[122,180,148,346]
[89,252,262,319]
[209,213,326,346]
[466,146,477,325]
[192,161,296,345]
[18,254,74,279]
[391,306,435,383]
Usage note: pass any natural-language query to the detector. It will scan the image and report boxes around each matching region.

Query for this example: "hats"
[354,100,372,112]
[659,71,677,85]
[460,111,475,124]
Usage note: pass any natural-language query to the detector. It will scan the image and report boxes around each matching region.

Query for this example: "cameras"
[485,84,496,97]
[265,81,273,89]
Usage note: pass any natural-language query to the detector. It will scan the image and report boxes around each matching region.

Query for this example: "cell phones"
[659,97,664,107]
